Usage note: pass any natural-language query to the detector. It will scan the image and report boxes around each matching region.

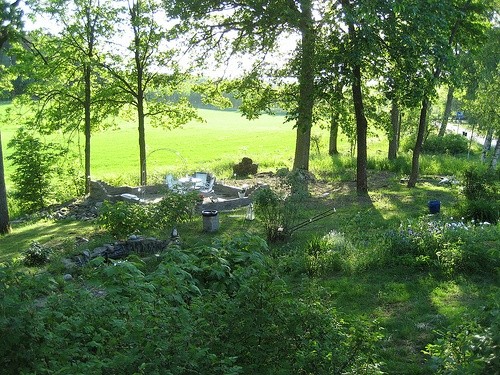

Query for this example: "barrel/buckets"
[202,210,219,233]
[428,200,440,213]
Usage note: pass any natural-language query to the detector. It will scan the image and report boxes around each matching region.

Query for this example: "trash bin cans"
[202,210,218,231]
